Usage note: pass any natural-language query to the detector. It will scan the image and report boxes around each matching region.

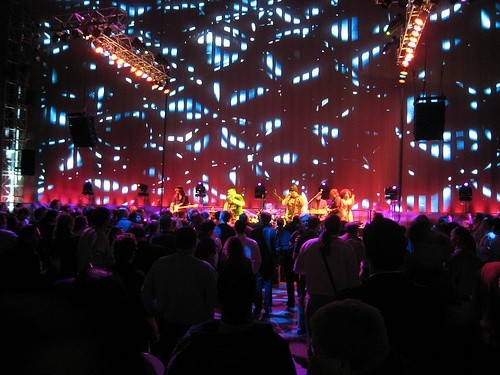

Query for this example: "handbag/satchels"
[335,289,359,301]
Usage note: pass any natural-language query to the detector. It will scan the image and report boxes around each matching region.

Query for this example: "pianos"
[308,209,327,214]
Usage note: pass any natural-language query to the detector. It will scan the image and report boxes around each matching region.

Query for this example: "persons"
[0,184,500,375]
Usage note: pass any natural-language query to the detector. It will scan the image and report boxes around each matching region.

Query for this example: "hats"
[343,222,361,231]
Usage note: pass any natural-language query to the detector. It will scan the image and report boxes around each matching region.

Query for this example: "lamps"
[449,0,461,5]
[385,185,398,200]
[255,183,266,199]
[196,181,206,198]
[83,179,93,196]
[137,183,150,196]
[382,0,439,82]
[459,182,472,203]
[319,181,330,200]
[11,20,171,96]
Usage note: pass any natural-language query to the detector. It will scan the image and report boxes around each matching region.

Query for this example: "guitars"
[170,202,199,214]
[274,190,298,214]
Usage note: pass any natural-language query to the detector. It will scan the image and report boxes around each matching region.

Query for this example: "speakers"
[414,97,445,141]
[67,112,98,148]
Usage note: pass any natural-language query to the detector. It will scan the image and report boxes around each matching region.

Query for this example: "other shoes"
[286,301,295,307]
[263,314,272,318]
[253,308,262,317]
